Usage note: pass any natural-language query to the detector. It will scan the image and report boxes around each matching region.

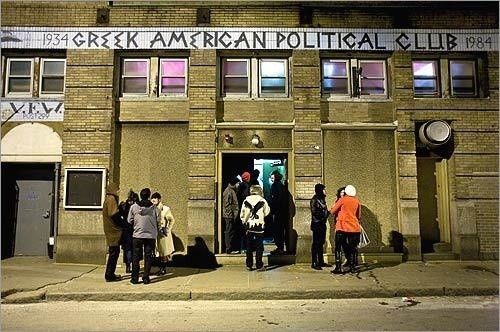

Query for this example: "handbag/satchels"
[357,226,370,248]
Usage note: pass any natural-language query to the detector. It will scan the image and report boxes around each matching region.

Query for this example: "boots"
[345,250,356,273]
[311,248,333,270]
[331,251,341,273]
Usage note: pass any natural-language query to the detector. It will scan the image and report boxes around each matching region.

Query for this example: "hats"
[242,172,250,182]
[250,185,261,194]
[345,185,356,197]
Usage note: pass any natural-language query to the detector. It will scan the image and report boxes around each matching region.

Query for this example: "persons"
[103,183,127,281]
[127,188,163,284]
[310,184,333,269]
[121,192,141,273]
[329,185,362,273]
[150,192,176,276]
[223,170,288,272]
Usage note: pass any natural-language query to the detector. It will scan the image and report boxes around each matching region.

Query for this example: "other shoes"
[227,248,231,254]
[257,267,264,271]
[143,277,151,284]
[104,275,121,282]
[130,279,137,284]
[126,270,132,275]
[246,267,252,270]
[269,248,284,254]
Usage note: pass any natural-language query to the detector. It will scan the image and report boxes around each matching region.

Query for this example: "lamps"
[251,135,260,145]
